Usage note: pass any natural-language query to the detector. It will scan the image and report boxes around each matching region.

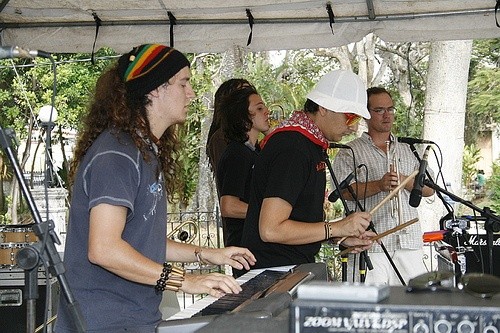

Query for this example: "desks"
[377,286,500,307]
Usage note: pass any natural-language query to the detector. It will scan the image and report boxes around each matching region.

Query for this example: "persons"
[471,170,485,188]
[51,43,256,333]
[205,70,433,285]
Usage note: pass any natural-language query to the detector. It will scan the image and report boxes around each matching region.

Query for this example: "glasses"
[369,107,397,115]
[344,113,361,127]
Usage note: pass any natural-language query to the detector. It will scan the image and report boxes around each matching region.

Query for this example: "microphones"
[397,137,435,145]
[330,143,350,150]
[0,45,50,59]
[328,164,363,202]
[409,146,430,208]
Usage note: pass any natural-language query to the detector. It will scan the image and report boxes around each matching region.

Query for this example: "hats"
[305,70,371,120]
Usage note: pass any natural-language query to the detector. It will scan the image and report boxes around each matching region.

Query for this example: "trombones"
[34,220,197,333]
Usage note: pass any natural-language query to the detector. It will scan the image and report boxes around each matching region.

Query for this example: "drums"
[0,224,39,270]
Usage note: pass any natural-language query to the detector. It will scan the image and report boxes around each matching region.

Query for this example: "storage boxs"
[0,264,59,333]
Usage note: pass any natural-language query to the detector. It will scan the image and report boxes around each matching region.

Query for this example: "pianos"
[155,262,328,333]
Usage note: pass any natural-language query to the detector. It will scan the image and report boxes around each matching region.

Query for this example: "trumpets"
[384,139,410,234]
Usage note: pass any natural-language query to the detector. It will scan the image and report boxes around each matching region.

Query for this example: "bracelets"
[195,246,212,265]
[155,263,185,293]
